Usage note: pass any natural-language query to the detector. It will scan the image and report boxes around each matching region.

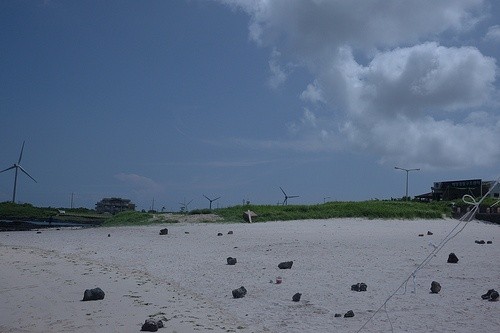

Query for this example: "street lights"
[394,166,421,207]
